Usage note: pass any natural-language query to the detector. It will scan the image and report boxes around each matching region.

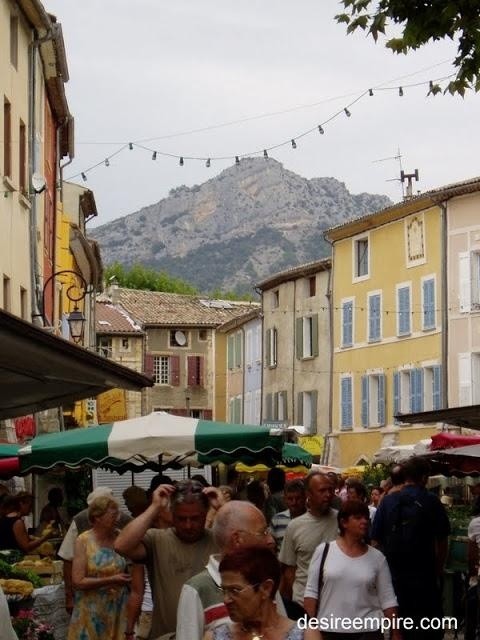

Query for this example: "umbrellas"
[0,411,314,487]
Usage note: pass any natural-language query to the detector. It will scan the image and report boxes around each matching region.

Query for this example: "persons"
[1,457,478,640]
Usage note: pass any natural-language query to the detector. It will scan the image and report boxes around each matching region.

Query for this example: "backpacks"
[388,494,427,550]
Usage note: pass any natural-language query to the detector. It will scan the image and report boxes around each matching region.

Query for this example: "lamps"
[34,270,95,345]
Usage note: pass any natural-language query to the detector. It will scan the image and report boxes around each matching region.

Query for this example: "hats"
[87,486,113,505]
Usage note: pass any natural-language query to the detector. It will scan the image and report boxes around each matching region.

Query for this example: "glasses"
[175,486,203,495]
[238,526,273,537]
[217,582,259,596]
